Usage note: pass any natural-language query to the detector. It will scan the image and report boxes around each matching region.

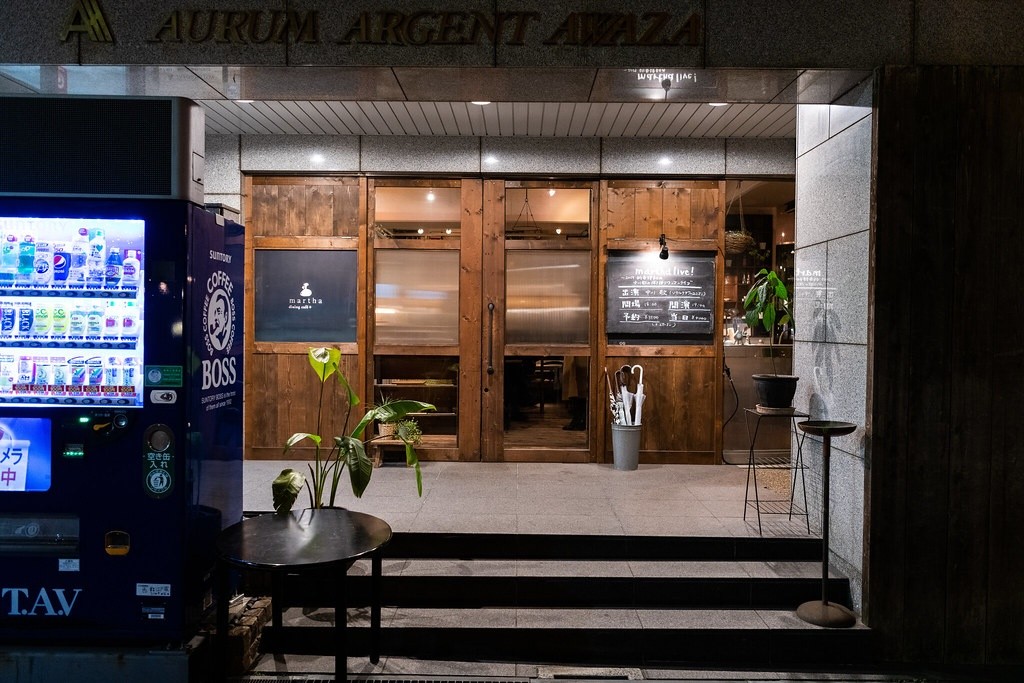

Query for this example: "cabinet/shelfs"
[743,407,811,535]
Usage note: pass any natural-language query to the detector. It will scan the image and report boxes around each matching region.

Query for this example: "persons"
[562,356,589,431]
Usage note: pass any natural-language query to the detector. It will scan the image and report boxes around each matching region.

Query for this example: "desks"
[212,508,393,683]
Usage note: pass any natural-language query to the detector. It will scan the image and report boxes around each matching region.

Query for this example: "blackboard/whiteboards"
[254,248,357,342]
[604,249,716,346]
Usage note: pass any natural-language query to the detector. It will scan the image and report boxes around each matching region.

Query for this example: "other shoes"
[562,420,586,431]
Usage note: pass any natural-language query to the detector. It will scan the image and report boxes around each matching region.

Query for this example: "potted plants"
[742,268,799,408]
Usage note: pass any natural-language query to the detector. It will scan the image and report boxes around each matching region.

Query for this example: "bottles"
[68,228,89,289]
[0,300,136,338]
[0,234,18,288]
[85,230,106,290]
[121,250,141,291]
[32,242,53,288]
[121,357,138,397]
[104,248,123,290]
[0,355,101,396]
[14,235,34,289]
[103,356,119,397]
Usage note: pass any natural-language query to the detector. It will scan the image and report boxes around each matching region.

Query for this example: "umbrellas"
[603,364,646,426]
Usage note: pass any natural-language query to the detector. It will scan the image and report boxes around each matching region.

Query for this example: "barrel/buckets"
[611,422,642,471]
